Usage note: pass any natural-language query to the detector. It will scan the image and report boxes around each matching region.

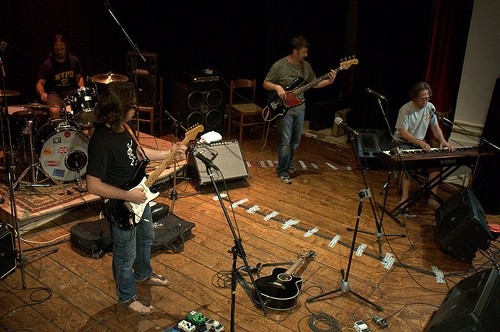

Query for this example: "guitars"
[251,249,315,311]
[267,54,359,120]
[99,123,204,231]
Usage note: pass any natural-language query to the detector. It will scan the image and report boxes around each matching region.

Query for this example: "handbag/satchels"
[70,218,112,258]
[153,213,195,253]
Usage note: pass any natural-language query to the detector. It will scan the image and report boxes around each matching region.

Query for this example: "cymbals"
[90,73,129,85]
[16,103,50,108]
[0,88,20,97]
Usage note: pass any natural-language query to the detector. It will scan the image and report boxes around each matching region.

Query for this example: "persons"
[35,34,84,120]
[86,81,188,316]
[263,38,337,184]
[393,83,454,213]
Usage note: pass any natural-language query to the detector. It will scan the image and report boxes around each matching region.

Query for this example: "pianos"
[380,145,497,217]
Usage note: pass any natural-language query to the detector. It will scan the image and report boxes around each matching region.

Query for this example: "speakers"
[434,186,495,263]
[423,267,500,332]
[125,51,160,104]
[186,140,249,188]
[0,218,16,280]
[181,70,226,140]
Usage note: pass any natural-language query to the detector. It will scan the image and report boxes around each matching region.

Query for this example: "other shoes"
[398,201,409,211]
[279,174,292,183]
[429,198,440,209]
[288,165,297,173]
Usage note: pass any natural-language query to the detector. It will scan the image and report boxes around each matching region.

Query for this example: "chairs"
[132,75,163,135]
[227,79,265,142]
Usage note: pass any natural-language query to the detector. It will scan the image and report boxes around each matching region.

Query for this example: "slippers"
[136,272,170,285]
[119,295,155,315]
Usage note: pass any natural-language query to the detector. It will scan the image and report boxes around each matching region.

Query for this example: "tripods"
[0,115,72,290]
[158,121,206,215]
[304,130,385,311]
[345,98,414,261]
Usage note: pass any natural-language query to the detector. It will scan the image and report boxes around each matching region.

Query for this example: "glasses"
[128,102,139,112]
[416,97,430,102]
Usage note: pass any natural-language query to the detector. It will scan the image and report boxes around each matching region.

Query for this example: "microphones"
[191,150,218,172]
[333,116,360,137]
[364,87,385,99]
[104,3,109,16]
[431,108,440,117]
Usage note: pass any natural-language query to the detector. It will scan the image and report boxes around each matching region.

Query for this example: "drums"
[2,105,47,155]
[62,86,98,126]
[32,118,91,182]
